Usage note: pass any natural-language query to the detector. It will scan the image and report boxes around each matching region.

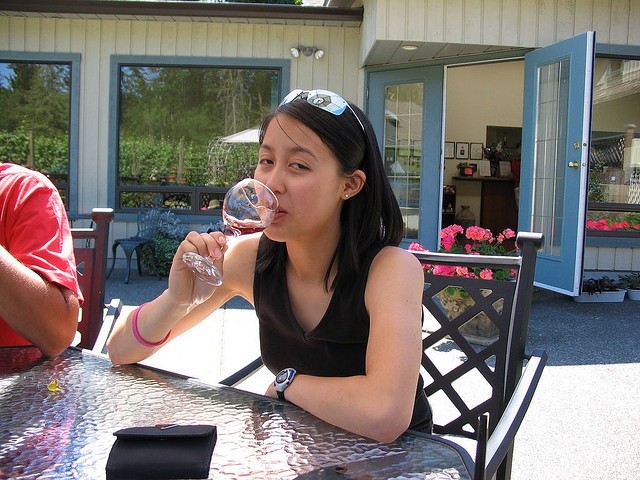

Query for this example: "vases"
[454,205,476,244]
[491,162,499,177]
[439,286,504,339]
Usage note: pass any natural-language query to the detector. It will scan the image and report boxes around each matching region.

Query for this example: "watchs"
[274,368,297,399]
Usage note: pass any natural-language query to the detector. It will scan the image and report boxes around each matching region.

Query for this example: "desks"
[1,344,476,479]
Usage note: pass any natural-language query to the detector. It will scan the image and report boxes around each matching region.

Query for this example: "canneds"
[159,176,166,186]
[180,178,187,186]
[167,176,176,187]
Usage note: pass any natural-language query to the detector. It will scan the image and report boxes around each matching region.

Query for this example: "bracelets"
[132,300,172,347]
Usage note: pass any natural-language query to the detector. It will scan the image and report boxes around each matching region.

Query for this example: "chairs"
[71,212,114,350]
[220,231,547,480]
[106,207,161,284]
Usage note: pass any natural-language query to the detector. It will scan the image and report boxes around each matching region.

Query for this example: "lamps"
[290,45,325,59]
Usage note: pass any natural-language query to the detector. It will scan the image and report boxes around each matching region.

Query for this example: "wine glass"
[182,178,278,287]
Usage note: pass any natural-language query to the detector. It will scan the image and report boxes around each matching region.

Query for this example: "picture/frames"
[398,139,412,156]
[456,142,469,159]
[445,142,455,159]
[413,140,422,157]
[470,143,483,160]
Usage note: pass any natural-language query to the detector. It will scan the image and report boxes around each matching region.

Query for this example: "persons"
[105,88,433,444]
[0,161,84,358]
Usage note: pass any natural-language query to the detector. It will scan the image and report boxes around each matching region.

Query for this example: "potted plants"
[618,273,640,301]
[572,276,627,303]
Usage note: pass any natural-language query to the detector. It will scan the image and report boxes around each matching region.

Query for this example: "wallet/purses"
[105,424,217,480]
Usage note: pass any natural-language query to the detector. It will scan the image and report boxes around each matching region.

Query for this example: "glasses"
[276,89,366,133]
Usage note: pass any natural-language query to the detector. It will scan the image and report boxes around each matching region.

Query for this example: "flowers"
[482,140,512,162]
[586,209,640,232]
[408,223,520,301]
